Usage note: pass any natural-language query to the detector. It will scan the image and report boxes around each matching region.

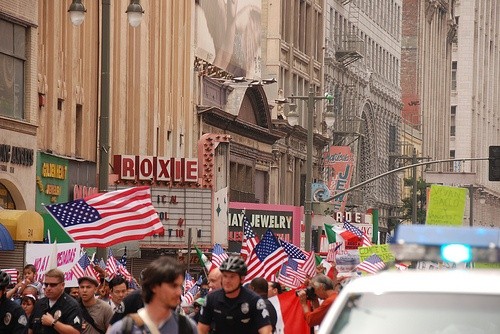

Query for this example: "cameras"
[32,318,40,326]
[295,287,316,301]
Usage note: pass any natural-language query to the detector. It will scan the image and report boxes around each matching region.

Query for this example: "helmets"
[0,271,10,291]
[219,256,247,276]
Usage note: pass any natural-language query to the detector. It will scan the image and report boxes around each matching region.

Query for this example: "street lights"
[67,0,144,269]
[397,148,432,224]
[287,94,335,254]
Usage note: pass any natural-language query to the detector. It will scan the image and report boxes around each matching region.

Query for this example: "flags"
[185,185,386,334]
[46,183,164,291]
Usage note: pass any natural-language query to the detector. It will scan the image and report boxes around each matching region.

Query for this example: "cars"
[319,270,500,334]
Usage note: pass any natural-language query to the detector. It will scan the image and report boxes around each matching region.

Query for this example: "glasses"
[44,281,63,288]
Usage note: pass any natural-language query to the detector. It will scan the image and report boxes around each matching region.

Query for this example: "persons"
[105,255,200,334]
[0,271,28,334]
[197,258,275,334]
[28,268,83,334]
[0,264,341,334]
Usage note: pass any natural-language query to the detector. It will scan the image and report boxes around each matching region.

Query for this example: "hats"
[78,277,99,287]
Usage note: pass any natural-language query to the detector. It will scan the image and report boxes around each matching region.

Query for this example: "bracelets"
[51,320,57,327]
